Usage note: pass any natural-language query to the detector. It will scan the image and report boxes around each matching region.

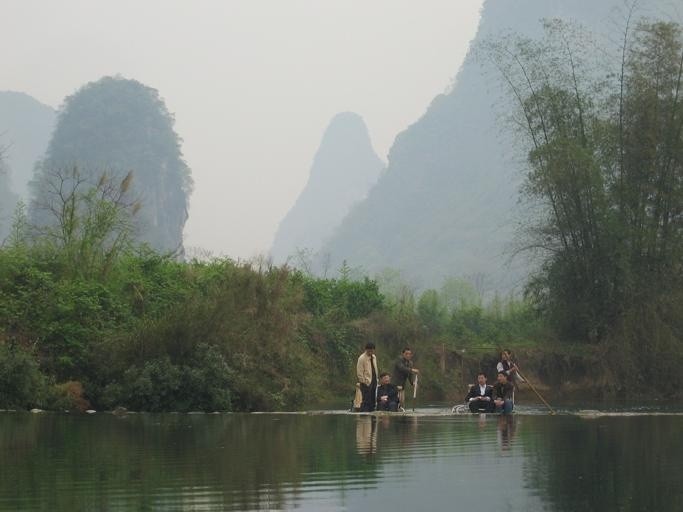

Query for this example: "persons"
[357,343,378,412]
[497,349,526,410]
[465,373,495,413]
[492,371,513,415]
[391,347,419,410]
[377,372,400,412]
